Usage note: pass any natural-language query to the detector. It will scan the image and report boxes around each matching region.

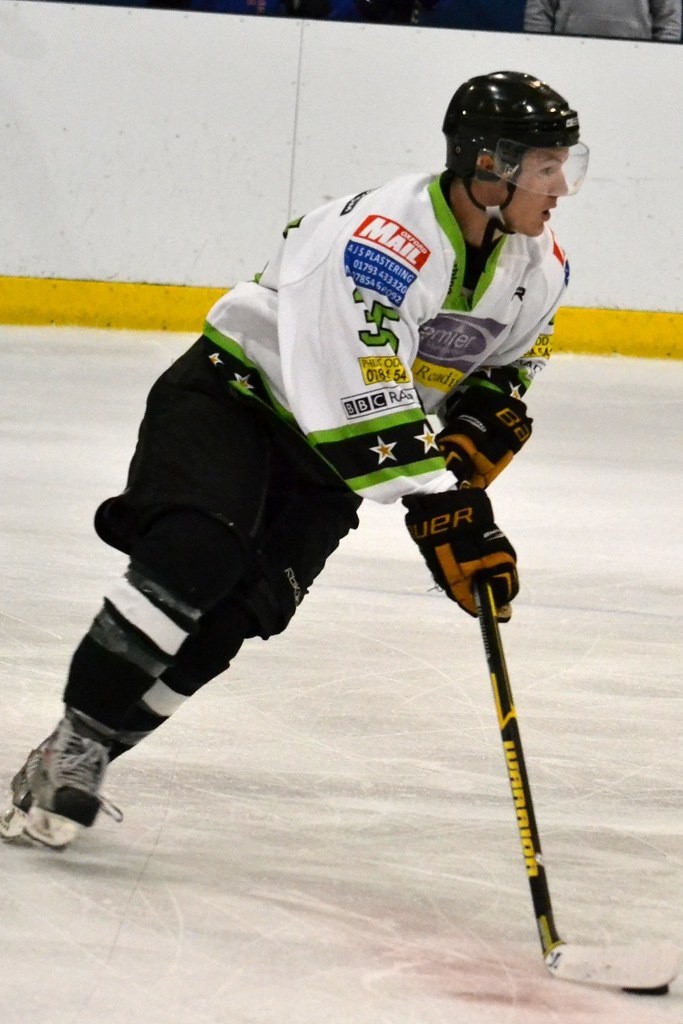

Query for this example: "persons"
[190,0,683,44]
[0,70,589,853]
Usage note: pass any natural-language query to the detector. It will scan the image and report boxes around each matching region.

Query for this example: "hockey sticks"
[476,575,679,989]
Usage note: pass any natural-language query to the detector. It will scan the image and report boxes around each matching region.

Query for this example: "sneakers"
[0,710,123,846]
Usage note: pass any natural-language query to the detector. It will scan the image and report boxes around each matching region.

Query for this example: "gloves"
[402,488,520,623]
[434,383,533,490]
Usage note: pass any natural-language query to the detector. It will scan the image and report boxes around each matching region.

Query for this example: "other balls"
[620,984,669,995]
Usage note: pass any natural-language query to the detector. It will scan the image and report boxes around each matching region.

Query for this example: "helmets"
[442,71,580,181]
